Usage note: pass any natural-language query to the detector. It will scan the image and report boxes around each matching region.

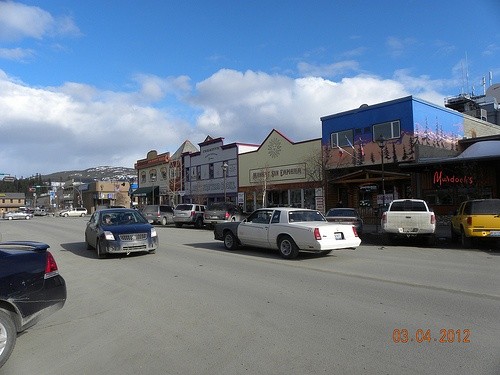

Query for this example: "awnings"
[398,140,500,171]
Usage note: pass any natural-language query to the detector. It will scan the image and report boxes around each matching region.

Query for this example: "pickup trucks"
[381,198,437,243]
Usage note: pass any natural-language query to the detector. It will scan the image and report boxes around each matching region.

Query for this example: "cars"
[214,206,362,259]
[0,239,67,370]
[2,206,55,220]
[84,207,159,259]
[324,207,365,234]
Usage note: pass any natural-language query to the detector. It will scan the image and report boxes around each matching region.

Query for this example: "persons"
[336,199,344,208]
[103,215,112,224]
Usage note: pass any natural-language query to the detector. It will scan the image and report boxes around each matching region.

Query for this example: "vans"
[203,202,248,230]
[172,203,207,229]
[137,203,176,226]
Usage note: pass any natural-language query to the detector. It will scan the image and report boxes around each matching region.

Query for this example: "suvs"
[59,206,88,217]
[451,199,500,250]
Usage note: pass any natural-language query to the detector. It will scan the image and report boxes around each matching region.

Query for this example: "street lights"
[375,135,387,206]
[220,162,229,202]
[151,174,156,205]
